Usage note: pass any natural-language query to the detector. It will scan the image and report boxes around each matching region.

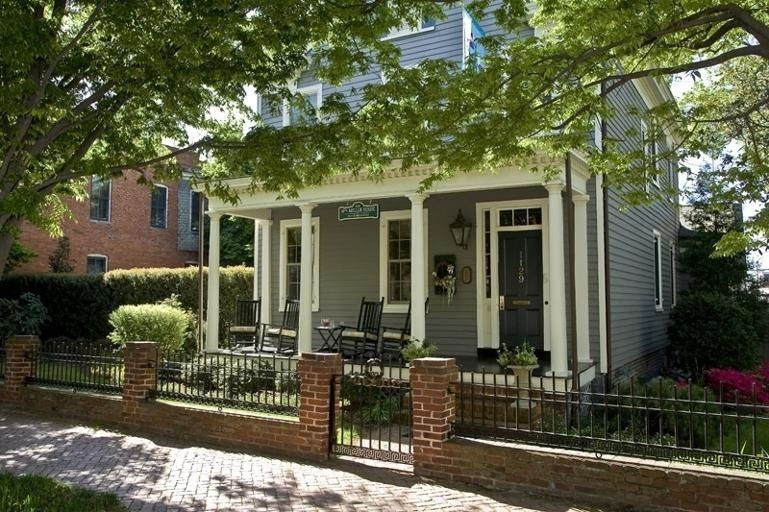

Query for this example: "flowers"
[431,259,457,304]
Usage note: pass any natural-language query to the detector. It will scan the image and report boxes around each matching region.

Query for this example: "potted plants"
[495,337,541,409]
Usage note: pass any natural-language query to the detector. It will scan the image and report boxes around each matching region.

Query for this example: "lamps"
[448,208,473,249]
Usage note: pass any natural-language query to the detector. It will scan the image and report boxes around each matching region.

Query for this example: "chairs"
[380,296,430,366]
[338,296,384,362]
[225,294,262,353]
[260,297,300,355]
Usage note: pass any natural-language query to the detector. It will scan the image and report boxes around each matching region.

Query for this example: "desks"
[314,324,342,353]
